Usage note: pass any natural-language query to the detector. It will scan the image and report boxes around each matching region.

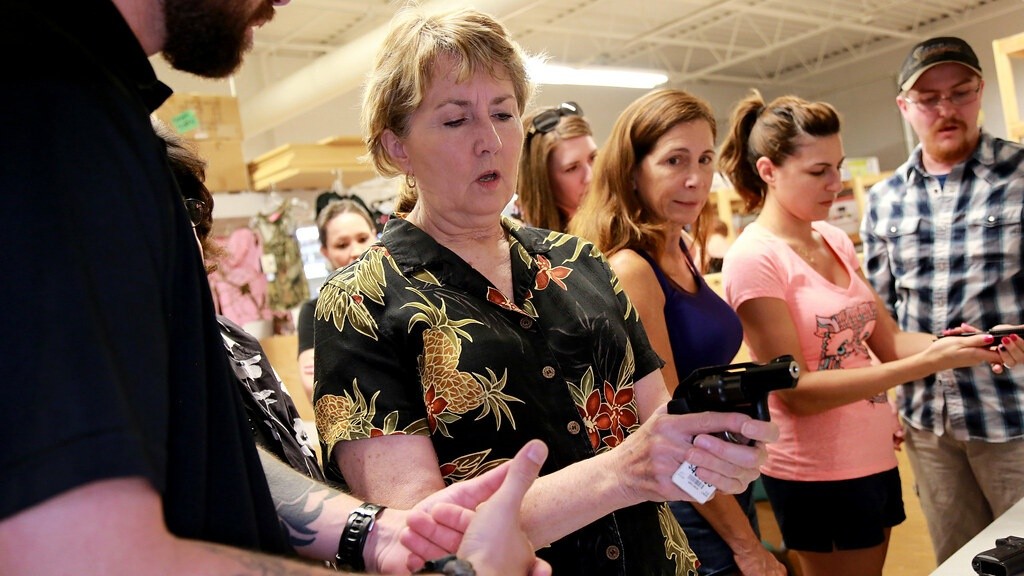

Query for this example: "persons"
[297,101,599,408]
[0,0,551,576]
[859,36,1024,566]
[723,89,1024,576]
[567,90,787,576]
[310,8,777,576]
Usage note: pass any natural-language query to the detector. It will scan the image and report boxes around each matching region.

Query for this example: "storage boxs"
[151,93,250,193]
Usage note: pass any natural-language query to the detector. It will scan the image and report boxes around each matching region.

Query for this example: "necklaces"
[794,246,816,264]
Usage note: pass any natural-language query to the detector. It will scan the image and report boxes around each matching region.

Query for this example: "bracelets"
[335,502,388,573]
[412,556,477,576]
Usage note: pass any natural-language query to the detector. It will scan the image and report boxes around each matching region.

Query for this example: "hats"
[901,81,982,112]
[897,36,983,93]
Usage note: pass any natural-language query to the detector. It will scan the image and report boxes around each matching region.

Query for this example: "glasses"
[522,101,584,153]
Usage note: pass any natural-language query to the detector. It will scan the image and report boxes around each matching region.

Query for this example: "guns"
[668,353,801,447]
[971,534,1024,575]
